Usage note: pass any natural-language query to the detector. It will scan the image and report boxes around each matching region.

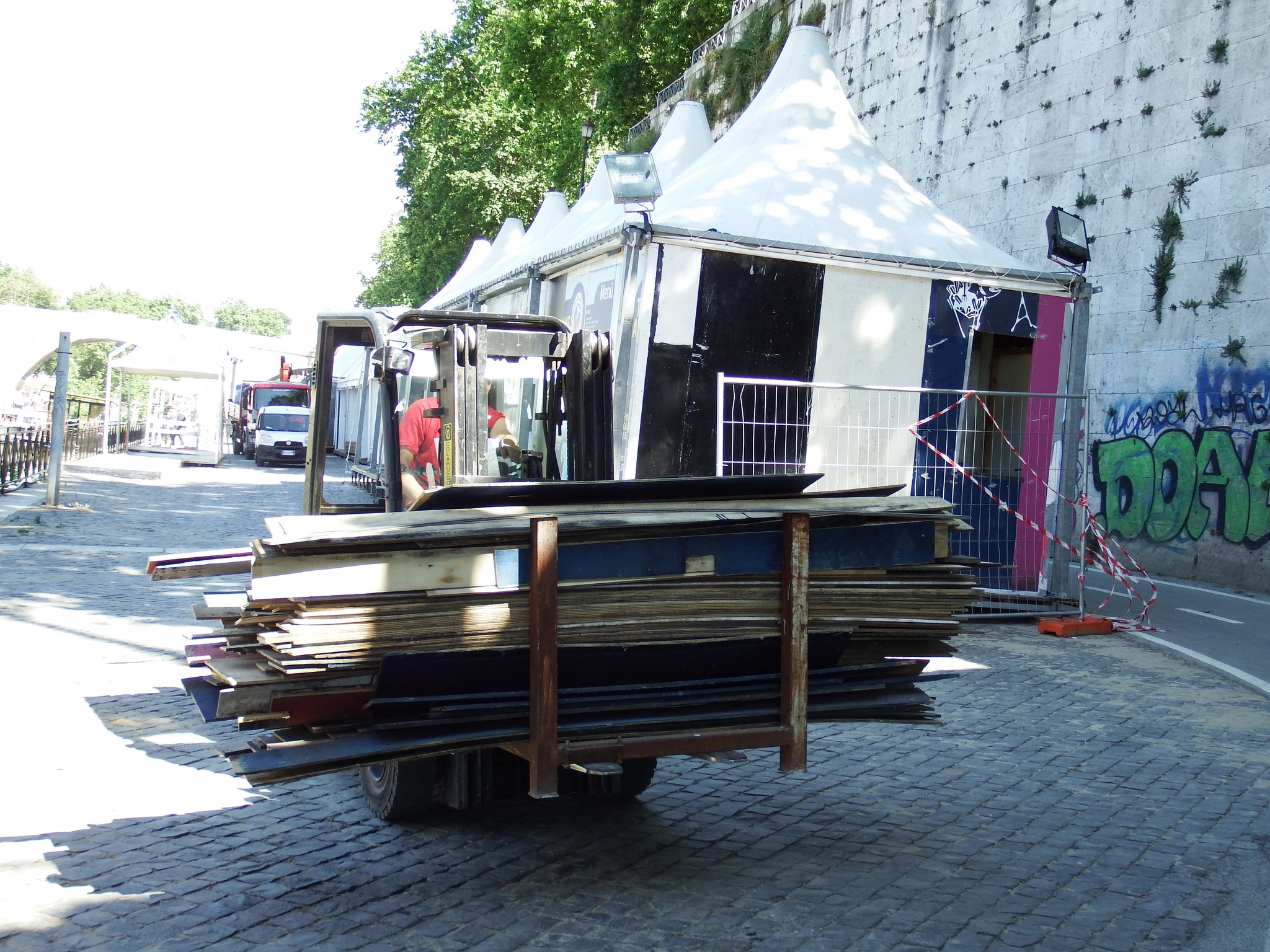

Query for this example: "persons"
[167,406,186,446]
[398,377,522,513]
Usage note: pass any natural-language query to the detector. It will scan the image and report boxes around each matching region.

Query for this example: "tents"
[102,25,1079,604]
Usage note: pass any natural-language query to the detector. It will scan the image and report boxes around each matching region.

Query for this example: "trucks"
[227,380,312,460]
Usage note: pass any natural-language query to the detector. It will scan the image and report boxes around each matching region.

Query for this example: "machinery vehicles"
[302,308,811,824]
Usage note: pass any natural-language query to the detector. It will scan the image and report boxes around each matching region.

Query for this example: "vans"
[248,406,310,467]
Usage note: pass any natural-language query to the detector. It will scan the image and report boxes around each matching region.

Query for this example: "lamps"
[604,152,662,248]
[1046,206,1090,299]
